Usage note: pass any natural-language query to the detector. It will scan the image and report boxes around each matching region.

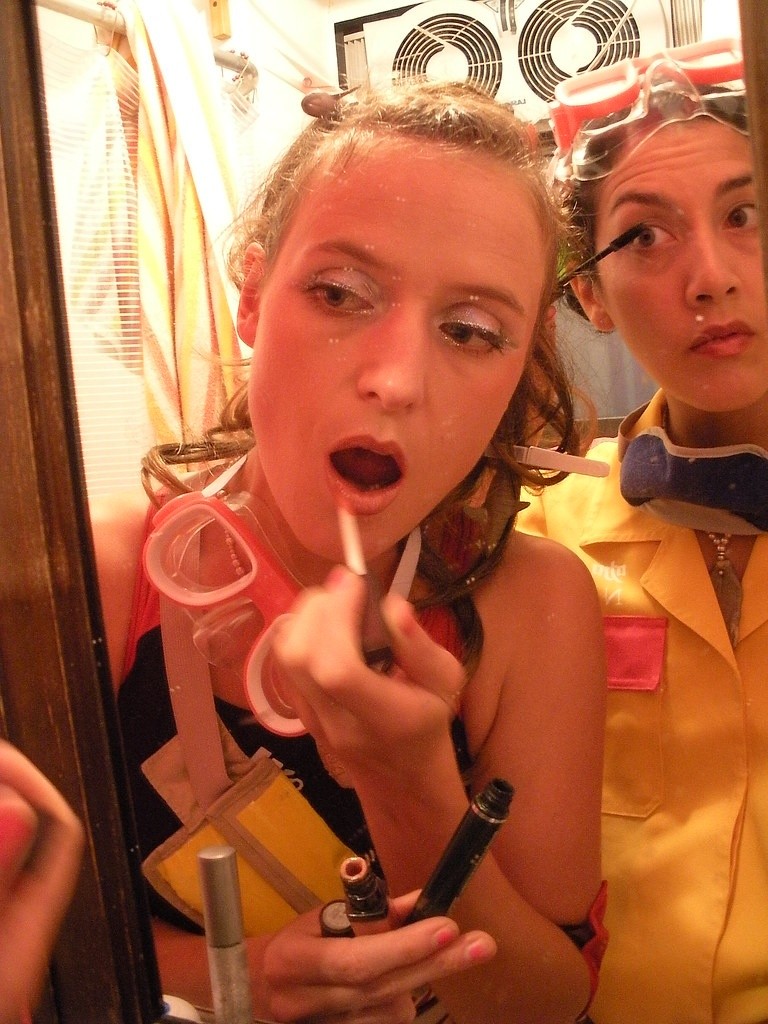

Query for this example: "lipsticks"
[334,502,391,655]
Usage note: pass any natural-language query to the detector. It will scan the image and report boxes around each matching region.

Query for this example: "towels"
[38,1,250,472]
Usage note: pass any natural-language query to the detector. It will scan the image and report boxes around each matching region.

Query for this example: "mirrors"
[19,0,768,1024]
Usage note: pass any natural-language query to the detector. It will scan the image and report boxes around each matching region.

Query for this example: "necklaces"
[216,490,429,590]
[706,531,744,649]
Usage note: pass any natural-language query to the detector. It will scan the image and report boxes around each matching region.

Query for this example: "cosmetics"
[319,778,515,939]
[195,845,256,1024]
[553,221,646,287]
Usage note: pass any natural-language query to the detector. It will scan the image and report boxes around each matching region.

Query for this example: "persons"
[515,82,768,1024]
[93,82,606,1024]
[0,739,86,1024]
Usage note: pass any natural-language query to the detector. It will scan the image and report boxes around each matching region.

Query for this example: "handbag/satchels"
[142,461,384,948]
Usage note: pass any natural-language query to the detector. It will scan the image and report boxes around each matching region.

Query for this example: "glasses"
[142,490,307,735]
[552,40,748,182]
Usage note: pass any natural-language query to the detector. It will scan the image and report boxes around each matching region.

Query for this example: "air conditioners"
[360,0,676,123]
